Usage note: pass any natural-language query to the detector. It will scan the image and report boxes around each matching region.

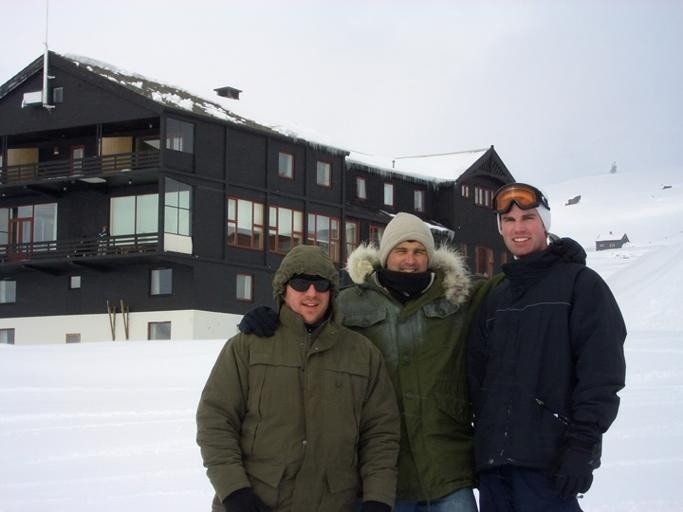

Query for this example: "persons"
[95,225,110,256]
[234,207,507,511]
[193,242,402,512]
[458,179,628,512]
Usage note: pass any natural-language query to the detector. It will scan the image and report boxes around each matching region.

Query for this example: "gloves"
[240,306,279,337]
[223,487,272,512]
[555,423,601,496]
[361,501,392,512]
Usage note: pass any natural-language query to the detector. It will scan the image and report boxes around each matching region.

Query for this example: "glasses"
[289,279,331,292]
[492,183,550,213]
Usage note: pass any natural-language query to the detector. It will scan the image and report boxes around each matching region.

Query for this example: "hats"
[497,183,551,236]
[379,212,435,269]
